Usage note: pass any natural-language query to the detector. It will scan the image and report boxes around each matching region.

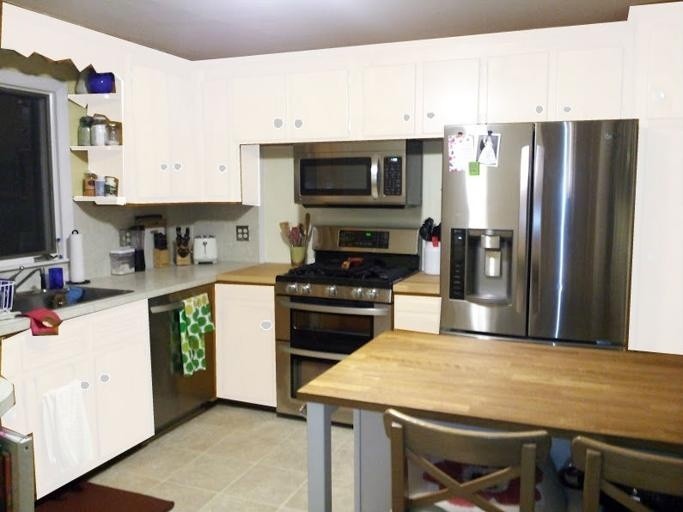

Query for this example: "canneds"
[77,117,92,146]
[82,173,97,196]
[108,123,119,146]
[91,117,108,145]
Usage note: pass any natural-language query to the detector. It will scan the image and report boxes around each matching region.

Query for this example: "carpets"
[35,479,174,512]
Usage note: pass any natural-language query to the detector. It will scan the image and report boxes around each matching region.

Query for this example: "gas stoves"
[276,261,417,289]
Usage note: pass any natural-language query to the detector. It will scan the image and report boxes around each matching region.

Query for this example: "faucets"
[9,266,45,293]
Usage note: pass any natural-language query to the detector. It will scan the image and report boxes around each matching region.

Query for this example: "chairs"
[381,409,567,512]
[559,434,683,512]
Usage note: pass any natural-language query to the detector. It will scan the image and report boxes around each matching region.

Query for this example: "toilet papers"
[70,233,84,284]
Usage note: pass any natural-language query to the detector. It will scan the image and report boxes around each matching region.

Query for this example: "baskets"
[0,280,14,313]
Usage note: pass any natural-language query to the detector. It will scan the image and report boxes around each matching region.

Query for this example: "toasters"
[194,235,218,265]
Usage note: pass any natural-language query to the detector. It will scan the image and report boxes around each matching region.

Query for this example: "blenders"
[120,225,145,271]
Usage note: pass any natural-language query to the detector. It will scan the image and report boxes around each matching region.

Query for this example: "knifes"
[176,226,190,257]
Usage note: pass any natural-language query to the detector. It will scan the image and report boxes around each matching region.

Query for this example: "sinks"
[13,287,135,312]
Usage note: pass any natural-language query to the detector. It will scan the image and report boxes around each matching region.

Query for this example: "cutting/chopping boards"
[139,218,167,270]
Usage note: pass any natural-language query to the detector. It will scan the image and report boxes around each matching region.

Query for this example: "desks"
[296,329,683,512]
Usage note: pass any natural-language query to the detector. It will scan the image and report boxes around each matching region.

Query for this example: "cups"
[421,239,441,276]
[291,246,306,265]
[49,267,64,289]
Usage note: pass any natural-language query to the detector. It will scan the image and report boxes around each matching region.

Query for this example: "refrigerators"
[439,117,640,351]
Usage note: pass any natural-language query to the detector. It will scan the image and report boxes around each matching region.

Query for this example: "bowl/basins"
[87,70,113,93]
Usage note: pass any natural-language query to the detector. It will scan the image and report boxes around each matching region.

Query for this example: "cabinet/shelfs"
[2,298,156,501]
[67,94,124,206]
[122,39,205,205]
[192,56,242,203]
[241,46,349,145]
[361,34,480,141]
[148,283,218,434]
[393,294,442,335]
[627,1,683,119]
[487,21,623,123]
[214,282,277,408]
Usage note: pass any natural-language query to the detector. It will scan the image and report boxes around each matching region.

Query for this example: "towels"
[179,292,215,376]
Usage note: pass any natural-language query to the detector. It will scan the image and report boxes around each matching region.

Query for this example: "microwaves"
[293,140,423,209]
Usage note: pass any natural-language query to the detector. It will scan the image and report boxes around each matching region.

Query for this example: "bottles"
[83,172,95,196]
[56,238,63,259]
[95,176,105,197]
[75,69,90,94]
[105,122,120,145]
[78,116,93,146]
[105,177,117,197]
[90,116,107,146]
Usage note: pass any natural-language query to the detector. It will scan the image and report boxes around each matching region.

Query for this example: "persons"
[477,135,497,165]
